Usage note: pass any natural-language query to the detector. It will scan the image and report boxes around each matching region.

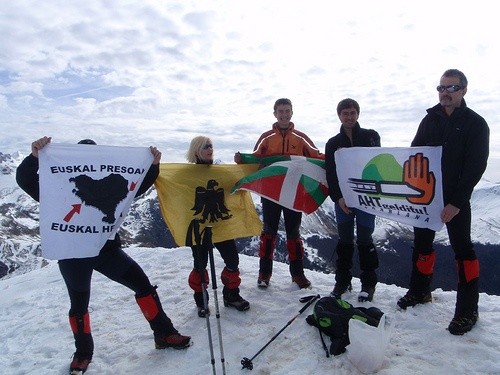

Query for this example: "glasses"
[203,144,213,149]
[437,85,465,93]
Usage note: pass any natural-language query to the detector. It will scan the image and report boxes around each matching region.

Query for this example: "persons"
[235,98,324,289]
[325,98,381,303]
[16,136,191,375]
[186,136,249,317]
[397,69,489,336]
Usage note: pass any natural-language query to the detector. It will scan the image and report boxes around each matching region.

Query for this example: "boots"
[221,266,250,311]
[69,309,94,375]
[134,284,191,350]
[188,268,211,317]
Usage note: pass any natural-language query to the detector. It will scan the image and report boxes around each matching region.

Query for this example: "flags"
[38,143,155,259]
[334,145,443,232]
[155,163,263,247]
[230,153,328,215]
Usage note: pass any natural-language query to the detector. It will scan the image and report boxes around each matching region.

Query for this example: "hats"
[77,139,97,145]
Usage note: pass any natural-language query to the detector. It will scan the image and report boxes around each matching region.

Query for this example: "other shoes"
[358,287,375,303]
[293,275,311,289]
[397,288,432,310]
[330,283,352,299]
[257,270,271,289]
[448,311,478,335]
